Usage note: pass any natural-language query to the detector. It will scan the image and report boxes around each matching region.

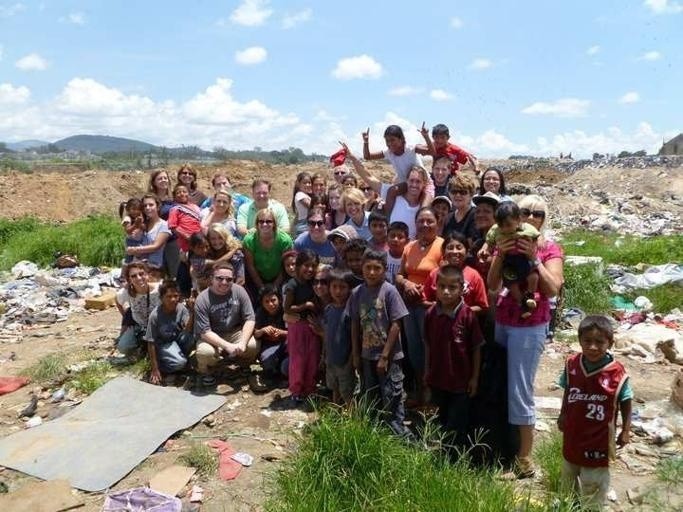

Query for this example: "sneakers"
[202,374,217,386]
[164,373,176,386]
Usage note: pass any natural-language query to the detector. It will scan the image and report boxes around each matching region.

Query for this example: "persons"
[557,315,634,511]
[116,122,565,481]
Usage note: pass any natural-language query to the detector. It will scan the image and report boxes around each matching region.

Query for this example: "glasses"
[215,276,233,282]
[519,208,545,220]
[334,171,345,174]
[308,221,323,227]
[451,189,468,196]
[362,187,372,190]
[130,272,144,278]
[256,220,272,225]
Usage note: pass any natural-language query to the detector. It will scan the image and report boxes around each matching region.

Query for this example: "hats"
[432,195,453,211]
[328,232,346,239]
[472,191,502,207]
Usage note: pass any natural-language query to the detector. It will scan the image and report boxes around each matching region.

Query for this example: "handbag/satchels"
[137,330,147,352]
